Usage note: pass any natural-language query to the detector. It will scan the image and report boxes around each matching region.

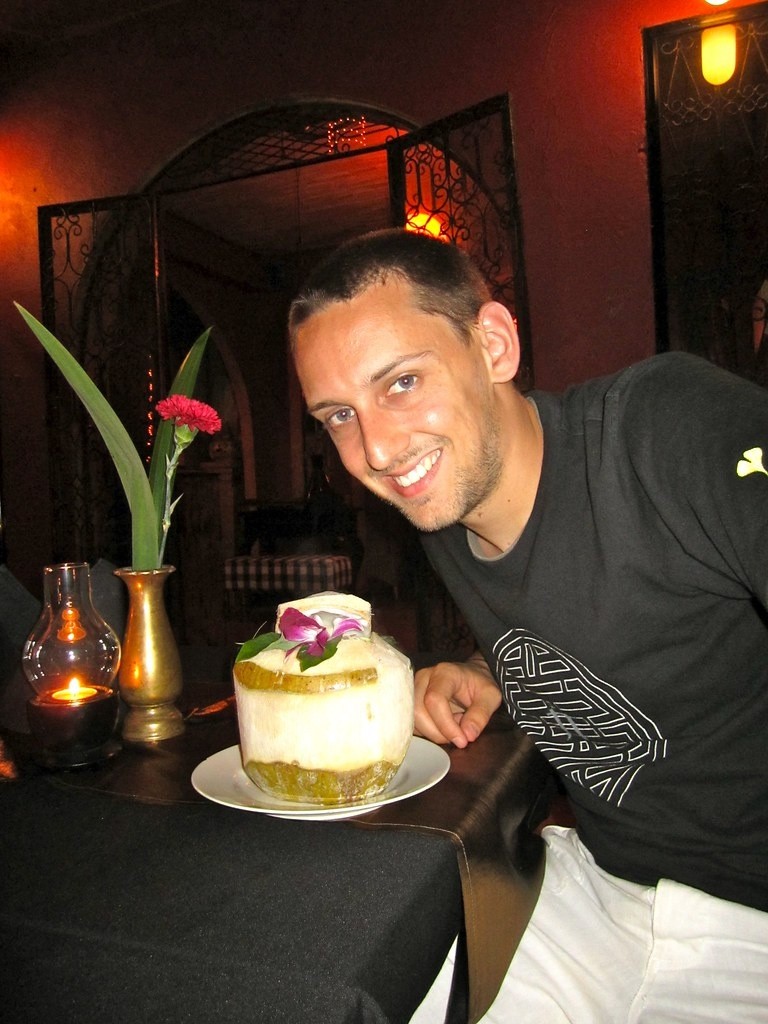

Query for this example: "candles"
[51,678,97,700]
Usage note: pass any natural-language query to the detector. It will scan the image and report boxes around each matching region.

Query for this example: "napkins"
[474,824,768,1023]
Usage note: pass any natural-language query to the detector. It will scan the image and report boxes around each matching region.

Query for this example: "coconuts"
[232,590,415,804]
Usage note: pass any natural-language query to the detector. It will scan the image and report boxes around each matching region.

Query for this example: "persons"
[287,228,768,1024]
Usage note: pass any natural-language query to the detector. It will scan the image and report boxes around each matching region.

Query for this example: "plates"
[190,734,451,820]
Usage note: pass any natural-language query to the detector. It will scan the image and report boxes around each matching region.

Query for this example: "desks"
[0,646,558,1024]
[225,556,352,606]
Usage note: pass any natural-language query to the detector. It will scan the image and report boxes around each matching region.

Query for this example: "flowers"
[236,607,363,673]
[12,300,222,571]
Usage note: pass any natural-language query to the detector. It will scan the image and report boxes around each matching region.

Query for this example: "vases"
[115,566,186,743]
